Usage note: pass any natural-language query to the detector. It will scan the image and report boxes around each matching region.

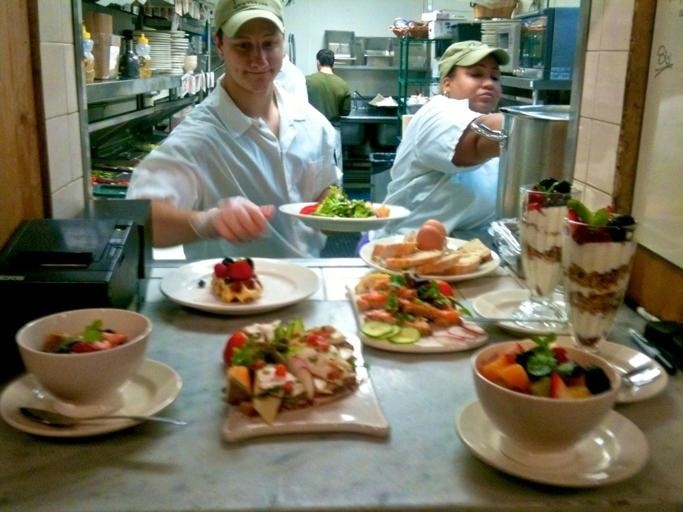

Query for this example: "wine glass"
[515,180,582,336]
[560,210,640,374]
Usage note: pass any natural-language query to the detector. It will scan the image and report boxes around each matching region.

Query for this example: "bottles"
[81,24,96,84]
[136,33,153,79]
[118,28,139,80]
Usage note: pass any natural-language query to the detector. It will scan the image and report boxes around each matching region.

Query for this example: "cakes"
[213,258,262,302]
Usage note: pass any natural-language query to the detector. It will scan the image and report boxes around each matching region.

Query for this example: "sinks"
[337,114,369,146]
[370,115,402,144]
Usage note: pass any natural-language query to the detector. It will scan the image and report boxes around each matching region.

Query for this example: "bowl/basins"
[466,337,624,472]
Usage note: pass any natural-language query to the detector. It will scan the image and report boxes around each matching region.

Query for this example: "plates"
[522,331,671,405]
[359,234,502,284]
[481,18,508,48]
[279,197,411,233]
[467,288,576,335]
[161,253,323,316]
[0,356,184,440]
[455,397,654,489]
[133,28,190,76]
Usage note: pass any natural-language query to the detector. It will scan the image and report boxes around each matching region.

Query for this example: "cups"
[184,55,197,75]
[189,35,203,54]
[14,308,154,421]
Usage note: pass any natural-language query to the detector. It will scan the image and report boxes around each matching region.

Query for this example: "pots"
[469,105,570,224]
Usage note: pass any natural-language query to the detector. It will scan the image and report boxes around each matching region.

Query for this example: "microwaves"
[492,21,527,74]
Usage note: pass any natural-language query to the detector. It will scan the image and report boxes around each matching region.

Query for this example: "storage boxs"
[420,12,465,39]
[87,30,125,80]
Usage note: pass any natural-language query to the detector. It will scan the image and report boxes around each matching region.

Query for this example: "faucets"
[354,89,367,100]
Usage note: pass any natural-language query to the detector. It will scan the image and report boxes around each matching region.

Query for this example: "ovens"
[513,4,580,82]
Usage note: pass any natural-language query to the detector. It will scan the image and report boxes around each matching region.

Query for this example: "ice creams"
[520,177,577,301]
[562,199,639,351]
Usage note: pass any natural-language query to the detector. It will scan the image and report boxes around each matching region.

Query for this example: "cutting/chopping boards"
[346,276,493,356]
[217,322,391,441]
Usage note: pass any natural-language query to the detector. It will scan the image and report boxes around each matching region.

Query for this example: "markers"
[626,327,678,376]
[625,294,660,322]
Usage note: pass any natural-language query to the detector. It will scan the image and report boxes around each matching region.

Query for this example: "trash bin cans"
[369,152,396,202]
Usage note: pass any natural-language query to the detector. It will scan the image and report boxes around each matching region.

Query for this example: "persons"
[274,51,309,102]
[355,38,505,259]
[306,49,352,195]
[125,0,344,261]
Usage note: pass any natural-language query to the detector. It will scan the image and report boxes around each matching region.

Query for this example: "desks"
[0,297,682,510]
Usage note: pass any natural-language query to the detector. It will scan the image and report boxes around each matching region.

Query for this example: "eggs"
[416,218,447,250]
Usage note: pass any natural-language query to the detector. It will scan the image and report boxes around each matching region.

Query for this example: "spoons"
[18,404,188,429]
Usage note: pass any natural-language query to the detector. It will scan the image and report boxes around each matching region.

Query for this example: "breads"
[372,237,490,277]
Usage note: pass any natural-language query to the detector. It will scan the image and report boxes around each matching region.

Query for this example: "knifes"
[460,311,571,325]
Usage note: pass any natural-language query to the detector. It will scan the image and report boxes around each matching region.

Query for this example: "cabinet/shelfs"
[397,32,446,143]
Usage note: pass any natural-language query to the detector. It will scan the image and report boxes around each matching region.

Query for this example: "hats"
[439,39,510,78]
[211,0,286,34]
